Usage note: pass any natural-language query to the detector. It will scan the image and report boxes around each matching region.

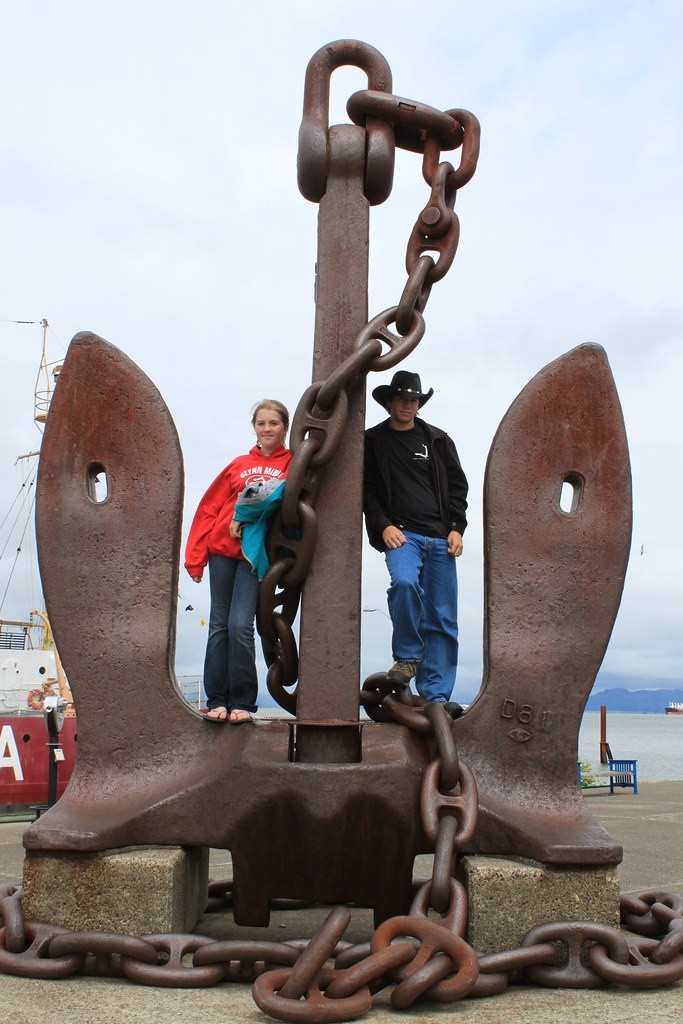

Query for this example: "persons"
[360,370,470,719]
[184,399,294,726]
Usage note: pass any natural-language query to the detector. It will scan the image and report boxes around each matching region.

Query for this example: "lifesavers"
[27,690,44,710]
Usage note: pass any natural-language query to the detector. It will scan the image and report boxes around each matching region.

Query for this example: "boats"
[663,702,683,716]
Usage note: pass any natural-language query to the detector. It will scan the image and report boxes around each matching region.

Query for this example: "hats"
[371,371,435,411]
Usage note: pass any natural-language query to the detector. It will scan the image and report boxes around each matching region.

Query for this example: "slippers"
[203,709,228,724]
[229,710,253,725]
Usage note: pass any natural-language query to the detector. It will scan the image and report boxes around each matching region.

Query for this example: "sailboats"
[0,318,232,815]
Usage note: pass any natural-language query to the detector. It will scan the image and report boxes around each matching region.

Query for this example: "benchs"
[605,743,637,794]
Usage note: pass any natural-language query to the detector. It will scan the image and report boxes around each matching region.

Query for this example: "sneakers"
[387,662,417,683]
[442,701,463,720]
